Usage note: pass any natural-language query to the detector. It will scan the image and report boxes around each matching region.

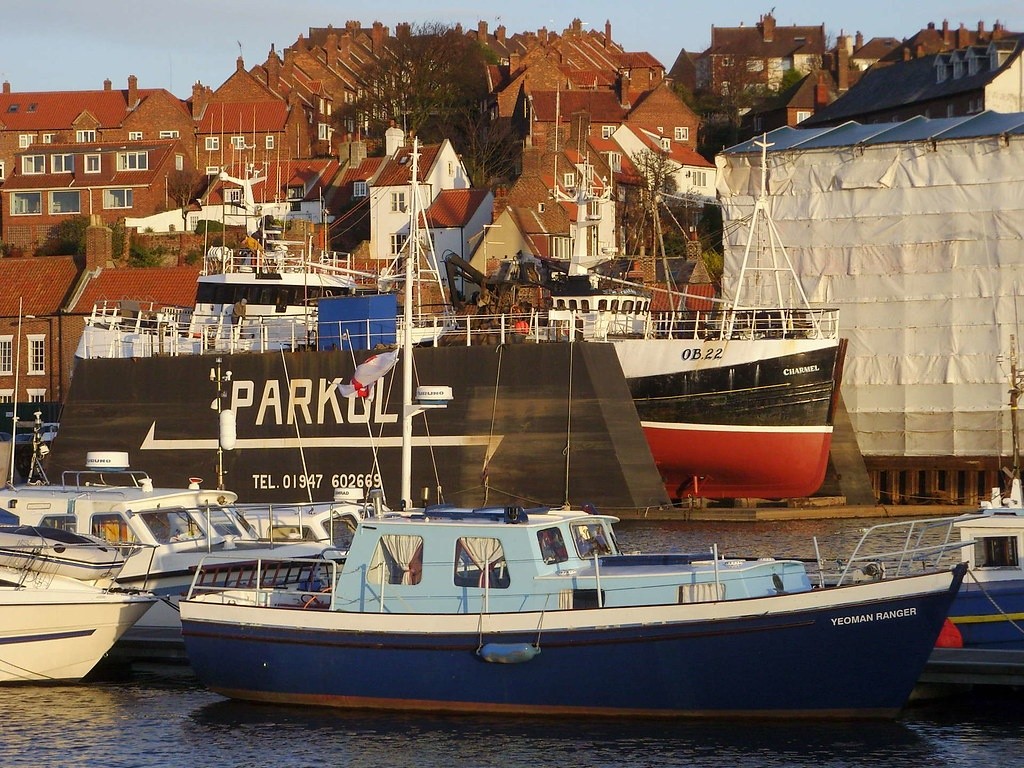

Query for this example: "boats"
[178,489,969,723]
[0,135,445,689]
[73,132,849,497]
[806,478,1024,686]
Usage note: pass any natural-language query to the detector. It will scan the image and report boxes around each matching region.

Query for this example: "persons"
[231,299,247,339]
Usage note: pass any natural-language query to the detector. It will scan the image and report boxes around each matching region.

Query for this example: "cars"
[0,425,59,443]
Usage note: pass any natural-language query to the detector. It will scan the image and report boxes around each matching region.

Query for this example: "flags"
[336,349,398,397]
